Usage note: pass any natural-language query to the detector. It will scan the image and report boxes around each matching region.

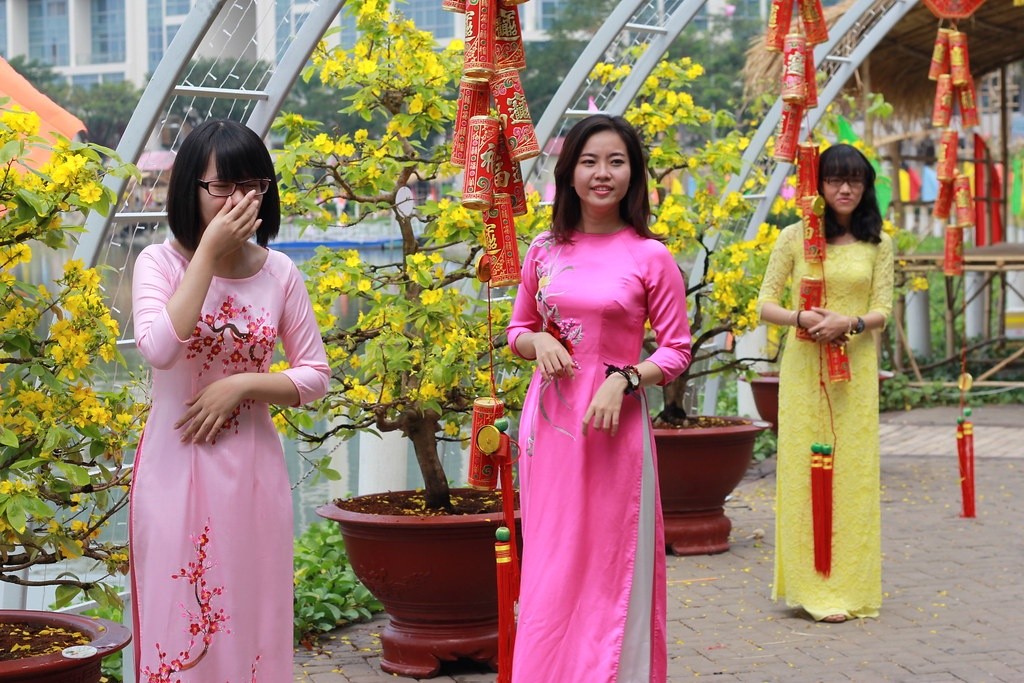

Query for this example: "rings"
[816,332,820,337]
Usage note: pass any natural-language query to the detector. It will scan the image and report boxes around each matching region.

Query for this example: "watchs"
[857,316,864,333]
[627,369,639,390]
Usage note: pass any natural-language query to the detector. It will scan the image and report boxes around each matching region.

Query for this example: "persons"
[756,145,895,623]
[129,122,331,683]
[505,115,692,683]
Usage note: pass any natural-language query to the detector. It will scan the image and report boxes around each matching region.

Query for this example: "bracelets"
[604,363,641,394]
[797,310,803,327]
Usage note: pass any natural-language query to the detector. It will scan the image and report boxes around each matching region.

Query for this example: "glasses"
[196,177,272,197]
[821,176,866,188]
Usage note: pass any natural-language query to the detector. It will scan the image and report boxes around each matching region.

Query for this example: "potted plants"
[0,97,155,683]
[260,0,555,680]
[586,42,774,556]
[741,68,929,437]
